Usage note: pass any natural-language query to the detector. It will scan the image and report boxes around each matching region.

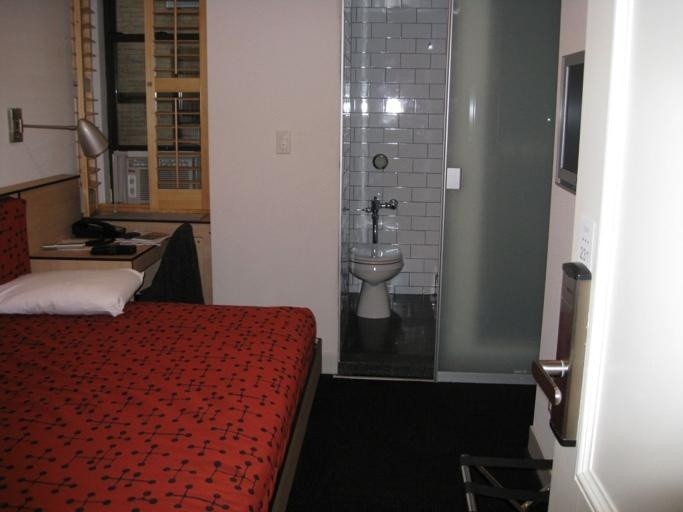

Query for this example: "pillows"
[0,269,144,317]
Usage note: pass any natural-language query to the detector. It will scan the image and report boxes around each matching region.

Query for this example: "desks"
[29,232,168,272]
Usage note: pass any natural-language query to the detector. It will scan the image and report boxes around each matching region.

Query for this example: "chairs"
[140,222,201,302]
[455,453,555,511]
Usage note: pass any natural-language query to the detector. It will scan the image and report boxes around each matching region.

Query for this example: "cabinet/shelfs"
[73,1,211,220]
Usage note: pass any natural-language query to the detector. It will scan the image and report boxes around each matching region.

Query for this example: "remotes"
[39,244,86,249]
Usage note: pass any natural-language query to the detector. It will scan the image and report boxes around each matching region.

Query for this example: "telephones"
[71,217,125,238]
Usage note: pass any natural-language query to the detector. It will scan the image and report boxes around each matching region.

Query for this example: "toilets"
[349,242,404,320]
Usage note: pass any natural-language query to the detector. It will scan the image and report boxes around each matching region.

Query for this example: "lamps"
[9,107,107,158]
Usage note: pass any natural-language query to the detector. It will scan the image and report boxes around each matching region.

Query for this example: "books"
[114,231,171,246]
[40,238,93,251]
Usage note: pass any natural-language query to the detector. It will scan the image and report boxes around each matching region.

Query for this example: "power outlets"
[274,129,290,155]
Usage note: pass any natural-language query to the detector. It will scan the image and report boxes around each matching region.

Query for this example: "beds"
[0,198,322,512]
